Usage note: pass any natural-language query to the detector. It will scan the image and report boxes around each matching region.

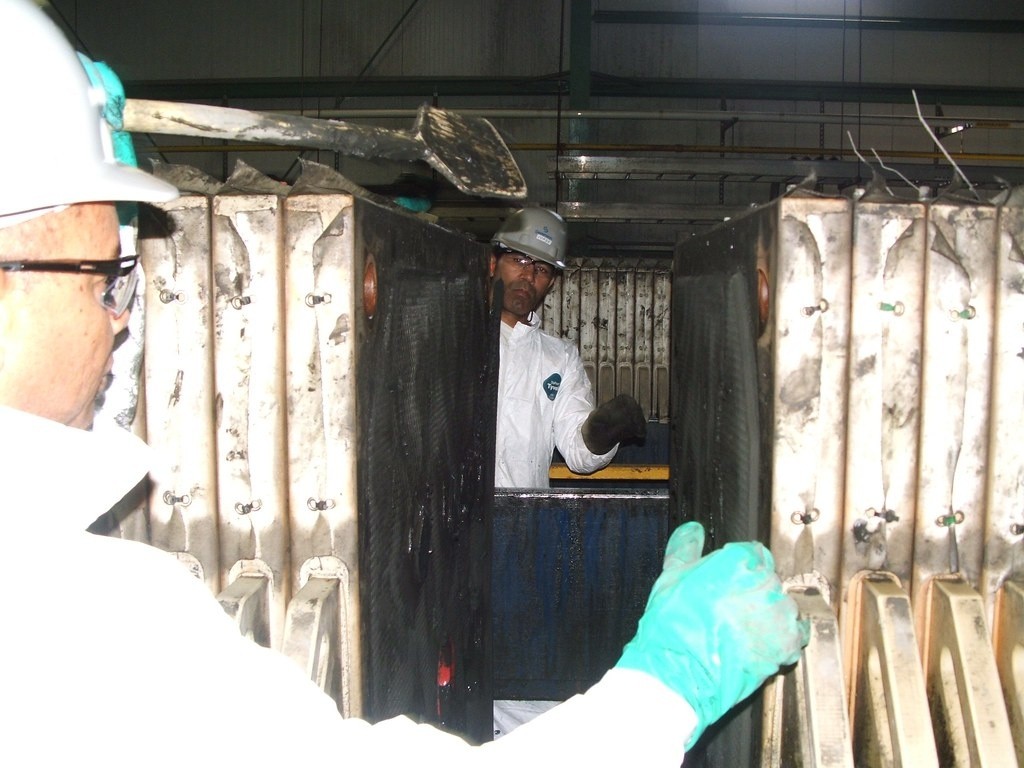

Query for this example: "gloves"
[615,522,810,752]
[581,394,647,455]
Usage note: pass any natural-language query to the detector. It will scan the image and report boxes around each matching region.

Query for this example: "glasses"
[0,254,140,320]
[503,250,556,279]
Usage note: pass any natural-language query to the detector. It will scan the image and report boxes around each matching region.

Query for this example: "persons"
[0,0,809,768]
[489,207,647,489]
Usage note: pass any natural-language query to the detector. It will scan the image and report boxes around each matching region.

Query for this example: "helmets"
[490,207,568,270]
[0,0,181,215]
